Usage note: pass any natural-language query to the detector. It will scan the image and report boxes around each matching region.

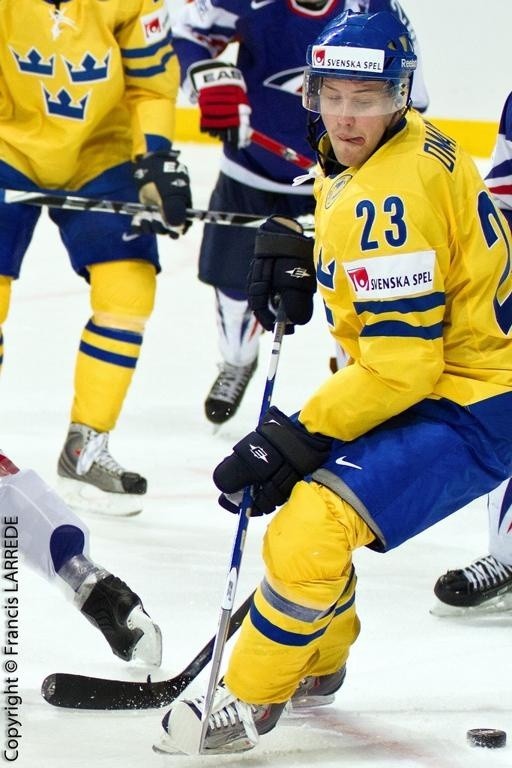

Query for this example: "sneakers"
[432,552,512,610]
[70,564,153,663]
[160,674,291,756]
[290,659,348,702]
[203,349,262,424]
[56,420,148,497]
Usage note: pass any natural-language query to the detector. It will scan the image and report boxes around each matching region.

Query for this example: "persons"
[164,13,512,758]
[169,1,423,423]
[434,84,511,606]
[1,1,194,498]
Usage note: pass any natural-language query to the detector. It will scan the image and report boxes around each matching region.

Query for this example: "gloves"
[119,148,195,244]
[212,403,336,519]
[243,213,318,336]
[186,55,254,153]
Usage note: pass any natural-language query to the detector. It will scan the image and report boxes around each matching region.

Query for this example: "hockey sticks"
[41,588,255,711]
[162,298,288,756]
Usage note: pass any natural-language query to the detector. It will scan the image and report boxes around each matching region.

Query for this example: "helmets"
[300,7,421,119]
